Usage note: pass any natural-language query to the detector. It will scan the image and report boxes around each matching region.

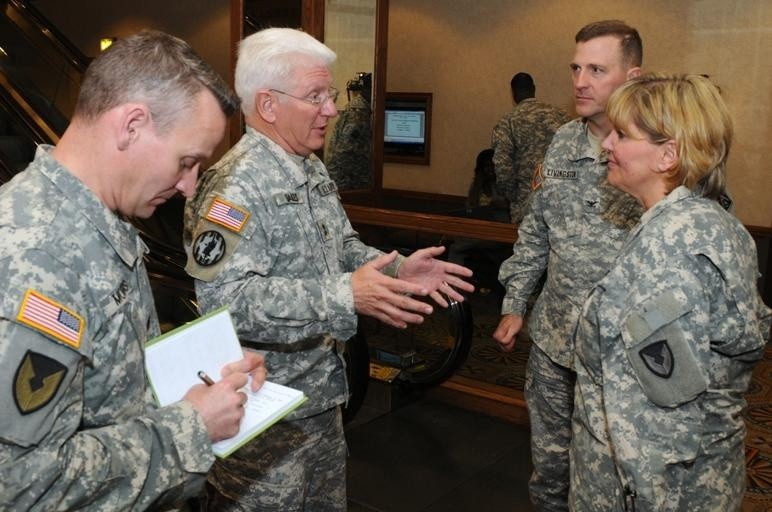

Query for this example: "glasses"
[270,87,339,109]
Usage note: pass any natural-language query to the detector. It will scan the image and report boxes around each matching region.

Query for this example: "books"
[143,305,309,460]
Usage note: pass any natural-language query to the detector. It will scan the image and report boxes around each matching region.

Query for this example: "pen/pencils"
[198,371,215,386]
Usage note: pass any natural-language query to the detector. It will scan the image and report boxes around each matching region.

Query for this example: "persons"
[0,28,267,512]
[492,19,644,512]
[564,72,772,511]
[490,72,573,339]
[323,70,372,191]
[466,124,506,209]
[182,27,476,512]
